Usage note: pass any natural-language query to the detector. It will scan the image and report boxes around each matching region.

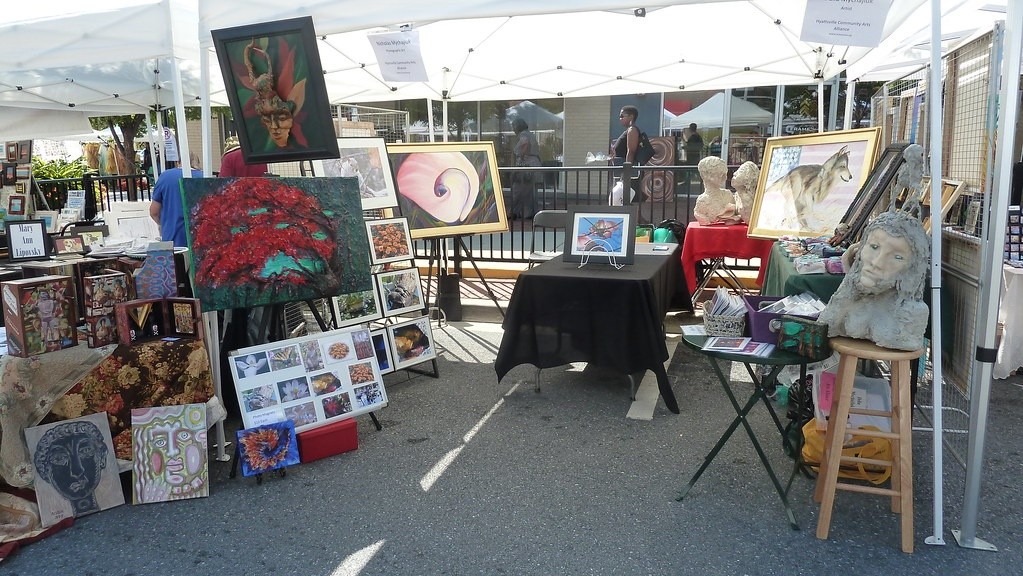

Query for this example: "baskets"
[702,299,745,337]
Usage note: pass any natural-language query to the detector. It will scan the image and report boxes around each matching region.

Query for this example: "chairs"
[529,210,568,269]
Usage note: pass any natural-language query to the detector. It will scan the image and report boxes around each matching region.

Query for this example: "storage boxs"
[742,295,784,344]
[0,254,204,359]
[298,419,359,464]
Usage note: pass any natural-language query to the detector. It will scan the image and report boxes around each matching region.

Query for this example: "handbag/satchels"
[783,375,816,461]
[803,418,898,485]
[608,181,635,207]
[630,126,656,166]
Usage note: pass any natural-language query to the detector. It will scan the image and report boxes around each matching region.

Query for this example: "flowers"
[234,354,266,378]
[322,396,346,416]
[312,374,338,396]
[281,379,311,403]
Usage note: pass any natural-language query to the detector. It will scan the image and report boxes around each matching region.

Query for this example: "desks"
[0,327,227,564]
[495,222,925,554]
[993,263,1023,381]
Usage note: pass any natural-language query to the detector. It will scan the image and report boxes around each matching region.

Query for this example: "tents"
[64,124,163,175]
[406,100,563,189]
[663,93,774,166]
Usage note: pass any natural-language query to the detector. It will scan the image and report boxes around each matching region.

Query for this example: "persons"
[504,119,545,219]
[610,106,645,224]
[673,122,767,180]
[822,212,931,351]
[140,141,176,185]
[150,151,203,281]
[695,157,758,224]
[219,136,269,177]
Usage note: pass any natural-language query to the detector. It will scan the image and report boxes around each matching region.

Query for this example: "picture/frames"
[0,139,109,262]
[210,15,509,435]
[746,126,882,240]
[562,205,639,265]
[830,143,915,249]
[911,177,966,236]
